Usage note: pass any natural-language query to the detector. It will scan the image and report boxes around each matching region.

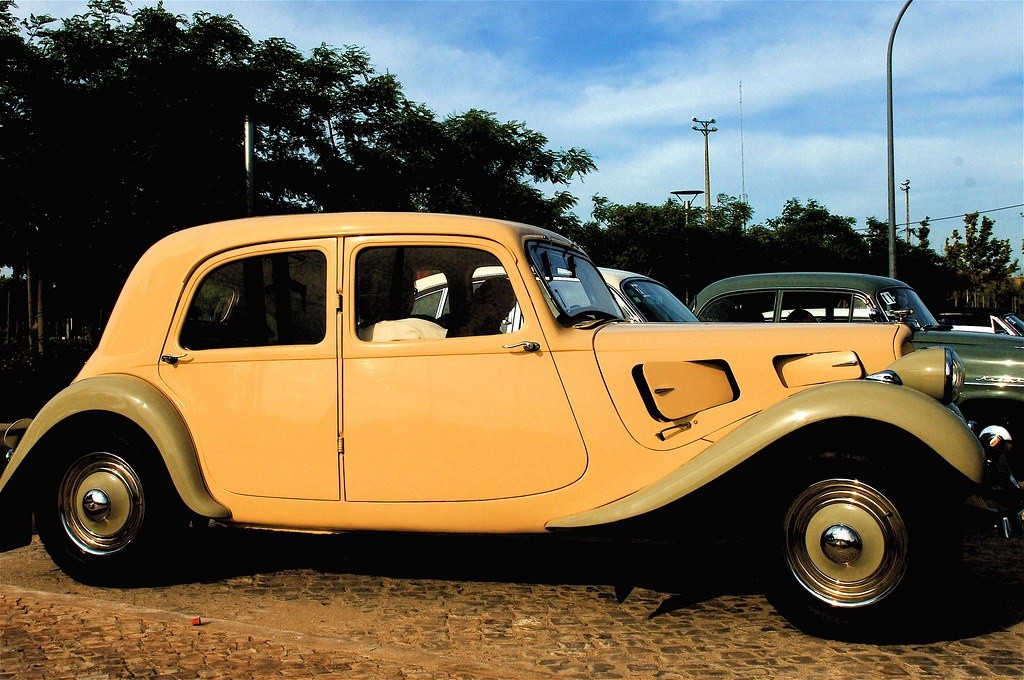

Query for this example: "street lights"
[900,179,910,246]
[692,116,719,227]
[670,189,705,263]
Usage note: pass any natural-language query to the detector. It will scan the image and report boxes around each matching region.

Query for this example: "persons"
[435,275,518,339]
[354,260,449,342]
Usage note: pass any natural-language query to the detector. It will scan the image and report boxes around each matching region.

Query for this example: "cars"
[2,212,1023,646]
[356,264,699,336]
[758,306,1023,337]
[687,270,1024,482]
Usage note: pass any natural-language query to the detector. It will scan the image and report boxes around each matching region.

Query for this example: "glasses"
[412,287,418,296]
